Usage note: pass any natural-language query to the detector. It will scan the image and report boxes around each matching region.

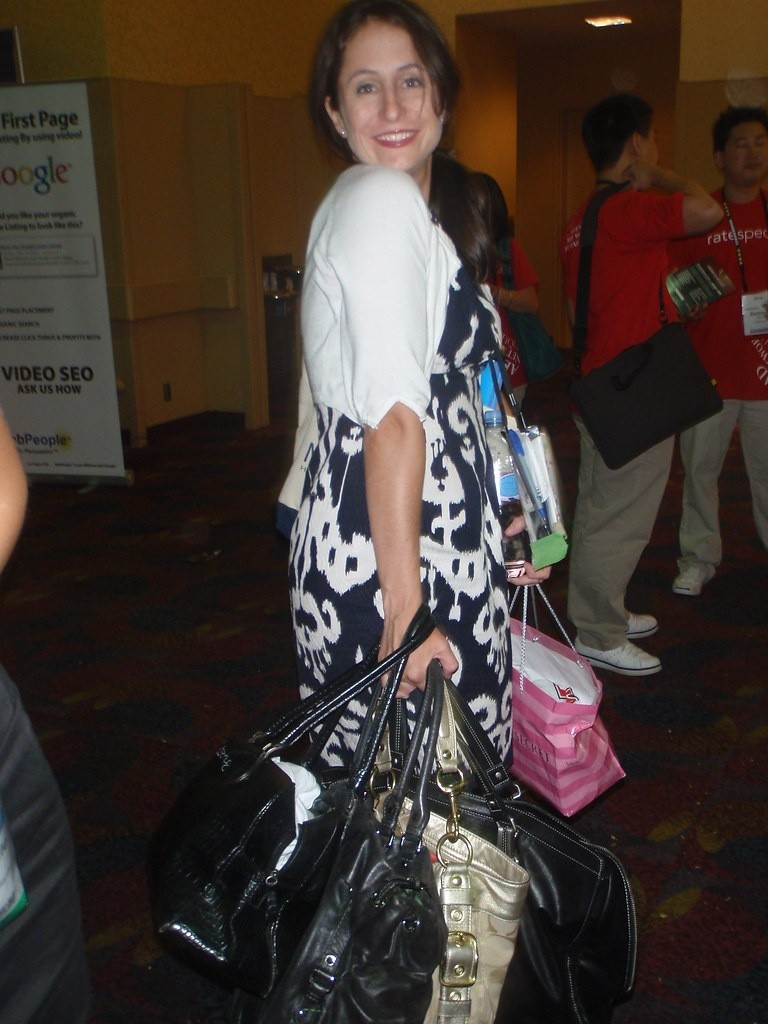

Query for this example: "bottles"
[498,499,532,579]
[484,410,518,510]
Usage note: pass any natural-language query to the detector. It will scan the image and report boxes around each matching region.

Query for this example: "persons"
[277,0,552,796]
[0,408,93,1024]
[470,172,539,411]
[559,95,724,677]
[671,106,768,595]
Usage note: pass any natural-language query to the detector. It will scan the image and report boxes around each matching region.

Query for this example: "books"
[666,257,736,320]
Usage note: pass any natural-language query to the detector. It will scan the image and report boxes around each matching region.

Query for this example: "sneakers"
[672,567,707,595]
[628,611,658,639]
[574,636,662,676]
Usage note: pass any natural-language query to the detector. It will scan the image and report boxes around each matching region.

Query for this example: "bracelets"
[504,290,513,308]
[493,288,499,299]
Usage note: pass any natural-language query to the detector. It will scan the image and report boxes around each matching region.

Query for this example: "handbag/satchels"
[143,607,638,1024]
[506,309,567,381]
[503,585,626,814]
[570,320,724,470]
